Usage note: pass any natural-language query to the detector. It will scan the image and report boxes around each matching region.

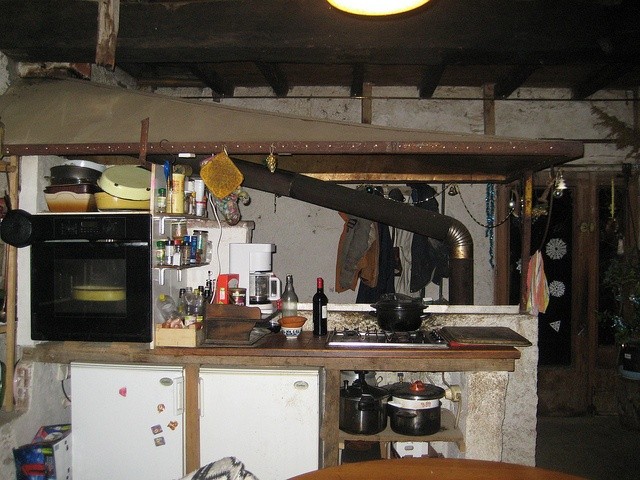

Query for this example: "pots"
[45,165,102,183]
[370,291,431,331]
[0,194,37,247]
[387,380,445,435]
[340,378,388,435]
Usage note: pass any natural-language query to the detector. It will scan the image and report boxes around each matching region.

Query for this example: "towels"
[525,251,550,317]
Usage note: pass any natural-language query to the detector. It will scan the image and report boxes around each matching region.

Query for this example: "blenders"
[229,242,281,315]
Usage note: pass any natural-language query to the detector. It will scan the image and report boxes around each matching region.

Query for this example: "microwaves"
[30,202,157,343]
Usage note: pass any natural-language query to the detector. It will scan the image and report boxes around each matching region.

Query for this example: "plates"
[44,193,95,212]
[97,163,151,201]
[45,182,102,194]
[95,193,150,210]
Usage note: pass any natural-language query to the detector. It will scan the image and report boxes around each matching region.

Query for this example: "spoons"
[1,278,6,323]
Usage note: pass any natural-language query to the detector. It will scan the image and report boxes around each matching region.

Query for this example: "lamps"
[448,188,455,196]
[508,197,516,208]
[553,175,568,198]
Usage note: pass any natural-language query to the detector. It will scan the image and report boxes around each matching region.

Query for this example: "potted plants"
[591,259,640,434]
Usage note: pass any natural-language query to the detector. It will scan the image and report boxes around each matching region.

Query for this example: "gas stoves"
[324,327,449,348]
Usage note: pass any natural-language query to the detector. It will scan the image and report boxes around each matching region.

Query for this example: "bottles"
[173,240,183,267]
[158,188,166,214]
[156,294,176,321]
[164,240,175,266]
[176,289,186,316]
[282,274,298,319]
[180,236,191,264]
[194,285,206,316]
[156,240,165,266]
[313,277,328,336]
[182,286,196,318]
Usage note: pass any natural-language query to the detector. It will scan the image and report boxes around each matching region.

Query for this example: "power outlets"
[450,386,461,403]
[57,363,71,381]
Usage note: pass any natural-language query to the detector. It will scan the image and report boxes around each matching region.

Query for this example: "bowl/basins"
[281,326,302,339]
[277,316,308,328]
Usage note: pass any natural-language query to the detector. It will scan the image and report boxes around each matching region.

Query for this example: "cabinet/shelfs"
[328,363,466,459]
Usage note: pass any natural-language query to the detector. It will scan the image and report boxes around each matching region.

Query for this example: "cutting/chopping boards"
[443,327,533,346]
